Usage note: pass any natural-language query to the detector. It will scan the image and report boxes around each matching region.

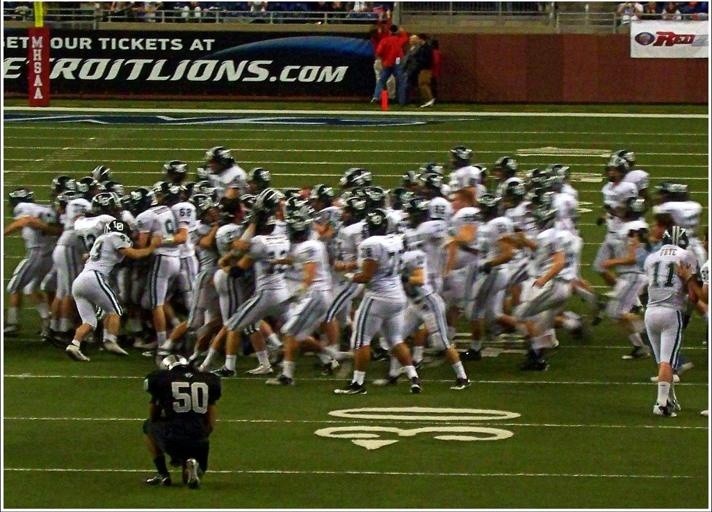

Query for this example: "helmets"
[388,161,445,228]
[160,354,189,370]
[626,197,647,213]
[663,226,688,248]
[48,165,149,215]
[104,219,132,237]
[151,148,281,236]
[606,149,635,170]
[479,155,570,230]
[450,148,473,167]
[337,167,373,190]
[7,188,35,209]
[282,182,388,241]
[660,182,688,196]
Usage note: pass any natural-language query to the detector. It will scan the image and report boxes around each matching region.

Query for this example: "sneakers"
[371,98,377,104]
[147,474,170,485]
[4,323,174,361]
[621,345,709,418]
[187,459,200,488]
[188,350,472,396]
[420,98,435,108]
[460,298,603,371]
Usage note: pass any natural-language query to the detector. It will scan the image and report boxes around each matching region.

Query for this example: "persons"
[641,225,700,419]
[140,352,222,490]
[365,21,442,113]
[4,2,393,25]
[5,139,707,396]
[614,2,709,31]
